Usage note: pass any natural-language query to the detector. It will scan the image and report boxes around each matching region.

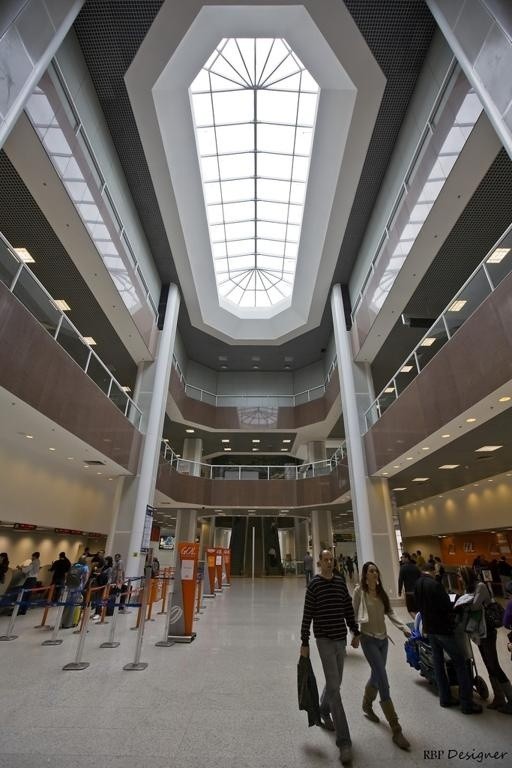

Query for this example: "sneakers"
[90,614,100,620]
[340,744,351,764]
[440,698,483,714]
[319,707,334,731]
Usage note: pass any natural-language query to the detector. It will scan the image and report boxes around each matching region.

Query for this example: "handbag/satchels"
[358,591,369,623]
[97,574,109,586]
[485,602,505,627]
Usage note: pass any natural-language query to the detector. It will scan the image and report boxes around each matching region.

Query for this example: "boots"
[379,699,409,747]
[487,677,512,714]
[363,683,379,722]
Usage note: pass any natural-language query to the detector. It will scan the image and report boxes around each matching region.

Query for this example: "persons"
[0,546,159,629]
[299,546,511,764]
[268,545,277,567]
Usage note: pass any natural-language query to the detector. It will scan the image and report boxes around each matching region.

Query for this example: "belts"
[362,631,395,646]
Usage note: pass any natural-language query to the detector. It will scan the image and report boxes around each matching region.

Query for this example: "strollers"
[407,600,490,704]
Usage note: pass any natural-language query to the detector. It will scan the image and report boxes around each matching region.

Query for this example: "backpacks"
[67,565,83,587]
